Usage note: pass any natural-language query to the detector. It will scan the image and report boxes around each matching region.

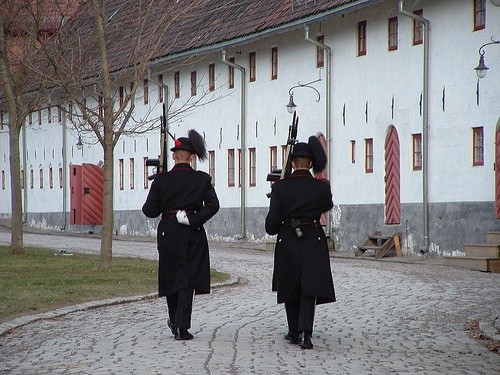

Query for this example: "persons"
[264,142,338,350]
[141,137,221,340]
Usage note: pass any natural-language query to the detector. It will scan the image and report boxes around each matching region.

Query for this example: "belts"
[282,218,320,228]
[163,210,196,219]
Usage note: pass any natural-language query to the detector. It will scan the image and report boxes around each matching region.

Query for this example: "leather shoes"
[175,328,194,340]
[284,331,298,344]
[167,318,175,335]
[298,332,313,348]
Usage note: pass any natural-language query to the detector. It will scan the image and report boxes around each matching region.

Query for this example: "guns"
[266,110,299,198]
[146,102,167,180]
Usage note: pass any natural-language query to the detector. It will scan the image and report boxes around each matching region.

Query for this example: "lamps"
[473,36,500,79]
[286,77,323,117]
[75,136,84,151]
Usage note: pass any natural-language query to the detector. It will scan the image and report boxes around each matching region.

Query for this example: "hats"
[292,143,316,156]
[170,137,197,152]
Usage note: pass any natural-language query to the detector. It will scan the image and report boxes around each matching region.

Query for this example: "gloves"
[176,210,190,226]
